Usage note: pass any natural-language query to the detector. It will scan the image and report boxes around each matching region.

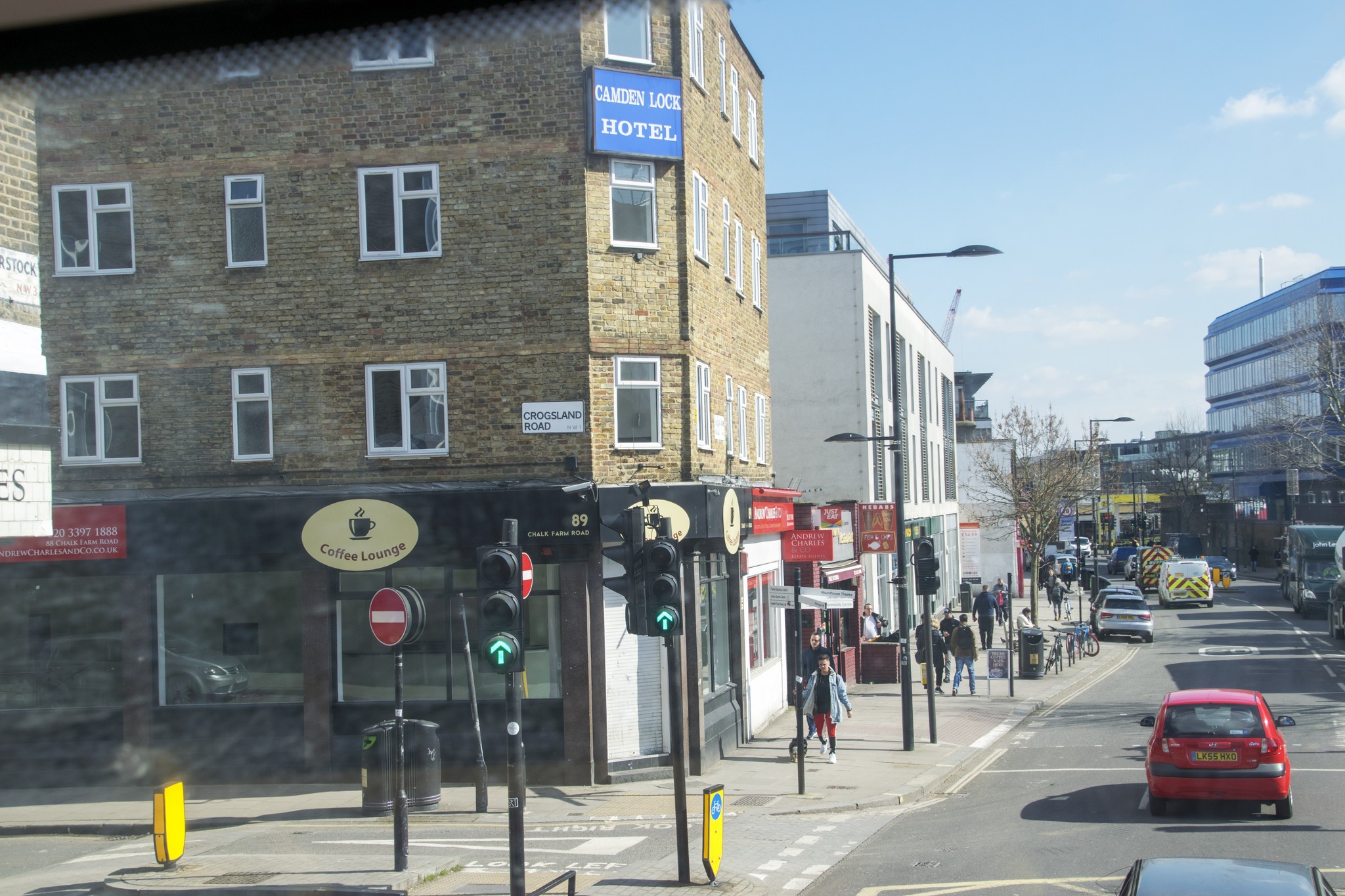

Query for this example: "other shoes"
[952,688,958,696]
[1059,617,1061,620]
[971,691,977,694]
[1048,604,1052,607]
[1055,616,1057,620]
[1043,638,1050,643]
[982,645,986,650]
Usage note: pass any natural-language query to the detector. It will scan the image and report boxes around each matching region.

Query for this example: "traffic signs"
[768,586,855,610]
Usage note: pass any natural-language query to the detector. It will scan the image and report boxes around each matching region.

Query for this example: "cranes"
[940,289,961,347]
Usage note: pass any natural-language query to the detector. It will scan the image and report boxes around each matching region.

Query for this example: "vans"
[1106,536,1214,609]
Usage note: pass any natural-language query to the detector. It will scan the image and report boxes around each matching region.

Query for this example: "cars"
[1115,688,1337,896]
[1202,556,1237,581]
[1123,555,1137,581]
[36,630,248,705]
[1025,537,1154,642]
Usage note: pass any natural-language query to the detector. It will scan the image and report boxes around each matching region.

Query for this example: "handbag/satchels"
[996,593,1003,606]
[915,650,926,665]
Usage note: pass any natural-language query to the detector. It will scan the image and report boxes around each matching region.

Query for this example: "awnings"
[820,562,864,584]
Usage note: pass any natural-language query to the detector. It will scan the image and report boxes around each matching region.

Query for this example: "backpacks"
[955,625,973,650]
[1065,562,1071,570]
[1052,585,1062,597]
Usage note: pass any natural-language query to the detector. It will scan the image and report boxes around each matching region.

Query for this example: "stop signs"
[522,553,533,600]
[370,587,408,646]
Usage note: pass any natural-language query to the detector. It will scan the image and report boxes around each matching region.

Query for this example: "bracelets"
[847,708,852,711]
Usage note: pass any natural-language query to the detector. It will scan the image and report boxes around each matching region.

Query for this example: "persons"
[972,584,1000,650]
[1062,558,1073,590]
[1017,607,1050,650]
[915,613,925,658]
[1049,578,1075,620]
[1038,557,1046,590]
[1130,540,1138,547]
[1043,565,1057,607]
[950,614,979,697]
[1146,539,1154,547]
[916,617,948,694]
[793,654,852,764]
[793,632,836,739]
[1248,546,1259,572]
[939,607,962,682]
[1274,547,1281,567]
[991,578,1008,626]
[863,603,888,641]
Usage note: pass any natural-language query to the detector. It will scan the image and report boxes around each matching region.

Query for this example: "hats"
[942,608,951,616]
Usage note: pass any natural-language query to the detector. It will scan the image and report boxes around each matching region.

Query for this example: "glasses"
[866,608,873,610]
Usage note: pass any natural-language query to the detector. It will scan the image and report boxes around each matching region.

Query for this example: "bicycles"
[1045,590,1100,675]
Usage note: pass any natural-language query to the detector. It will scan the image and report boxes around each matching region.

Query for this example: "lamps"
[751,486,764,494]
[707,488,720,496]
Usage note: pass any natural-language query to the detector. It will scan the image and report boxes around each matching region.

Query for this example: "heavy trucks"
[1282,524,1345,639]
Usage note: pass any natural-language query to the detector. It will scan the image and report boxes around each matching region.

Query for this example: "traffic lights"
[643,538,684,636]
[912,537,941,595]
[600,506,647,636]
[1101,523,1105,530]
[1111,515,1116,530]
[1131,514,1160,529]
[477,543,523,673]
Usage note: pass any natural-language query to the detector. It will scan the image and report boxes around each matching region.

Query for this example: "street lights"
[1040,417,1144,643]
[824,244,1003,751]
[1171,458,1253,573]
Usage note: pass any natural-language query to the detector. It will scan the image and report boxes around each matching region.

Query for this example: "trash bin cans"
[1018,627,1045,680]
[1091,575,1111,611]
[1081,568,1094,591]
[361,719,441,817]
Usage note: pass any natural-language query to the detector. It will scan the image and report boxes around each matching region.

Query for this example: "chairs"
[1224,719,1246,728]
[1230,708,1254,724]
[1003,619,1020,651]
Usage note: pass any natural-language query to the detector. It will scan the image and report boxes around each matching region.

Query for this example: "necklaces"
[820,671,829,684]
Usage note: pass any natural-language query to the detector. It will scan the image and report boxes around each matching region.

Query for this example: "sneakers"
[935,687,945,694]
[829,753,836,763]
[807,731,817,739]
[820,738,828,754]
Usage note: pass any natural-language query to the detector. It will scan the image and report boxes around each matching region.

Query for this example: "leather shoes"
[999,623,1002,626]
[943,677,950,683]
[960,676,962,681]
